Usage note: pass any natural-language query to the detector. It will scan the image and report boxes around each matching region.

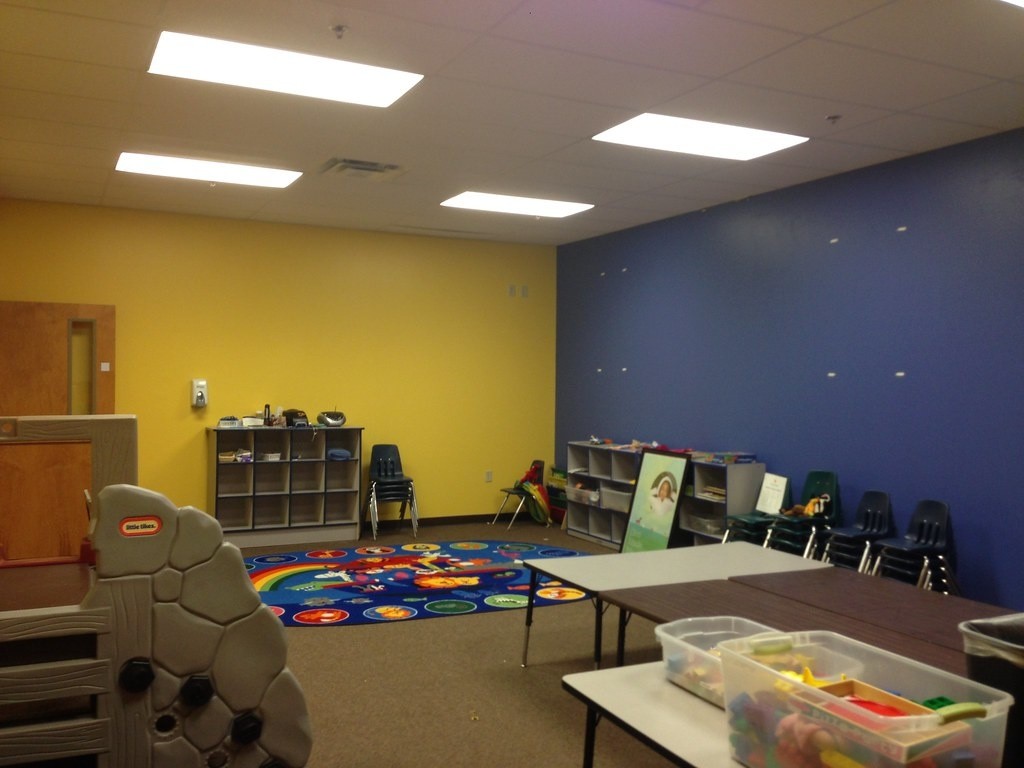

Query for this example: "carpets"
[243,540,594,627]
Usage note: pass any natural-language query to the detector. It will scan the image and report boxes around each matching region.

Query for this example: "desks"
[521,541,1020,768]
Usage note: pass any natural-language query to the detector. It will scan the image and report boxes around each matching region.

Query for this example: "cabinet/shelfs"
[205,426,366,549]
[566,441,766,553]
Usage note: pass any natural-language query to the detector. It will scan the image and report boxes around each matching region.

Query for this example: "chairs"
[819,491,895,576]
[492,459,545,531]
[870,498,962,596]
[721,473,794,548]
[763,471,844,560]
[363,444,418,540]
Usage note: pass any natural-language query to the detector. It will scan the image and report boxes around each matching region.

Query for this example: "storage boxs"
[564,485,601,507]
[654,615,782,712]
[718,630,1015,768]
[546,467,568,524]
[600,486,633,513]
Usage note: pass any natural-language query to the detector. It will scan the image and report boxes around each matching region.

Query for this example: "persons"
[650,476,675,512]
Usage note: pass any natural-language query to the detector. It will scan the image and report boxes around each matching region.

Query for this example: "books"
[697,484,726,502]
